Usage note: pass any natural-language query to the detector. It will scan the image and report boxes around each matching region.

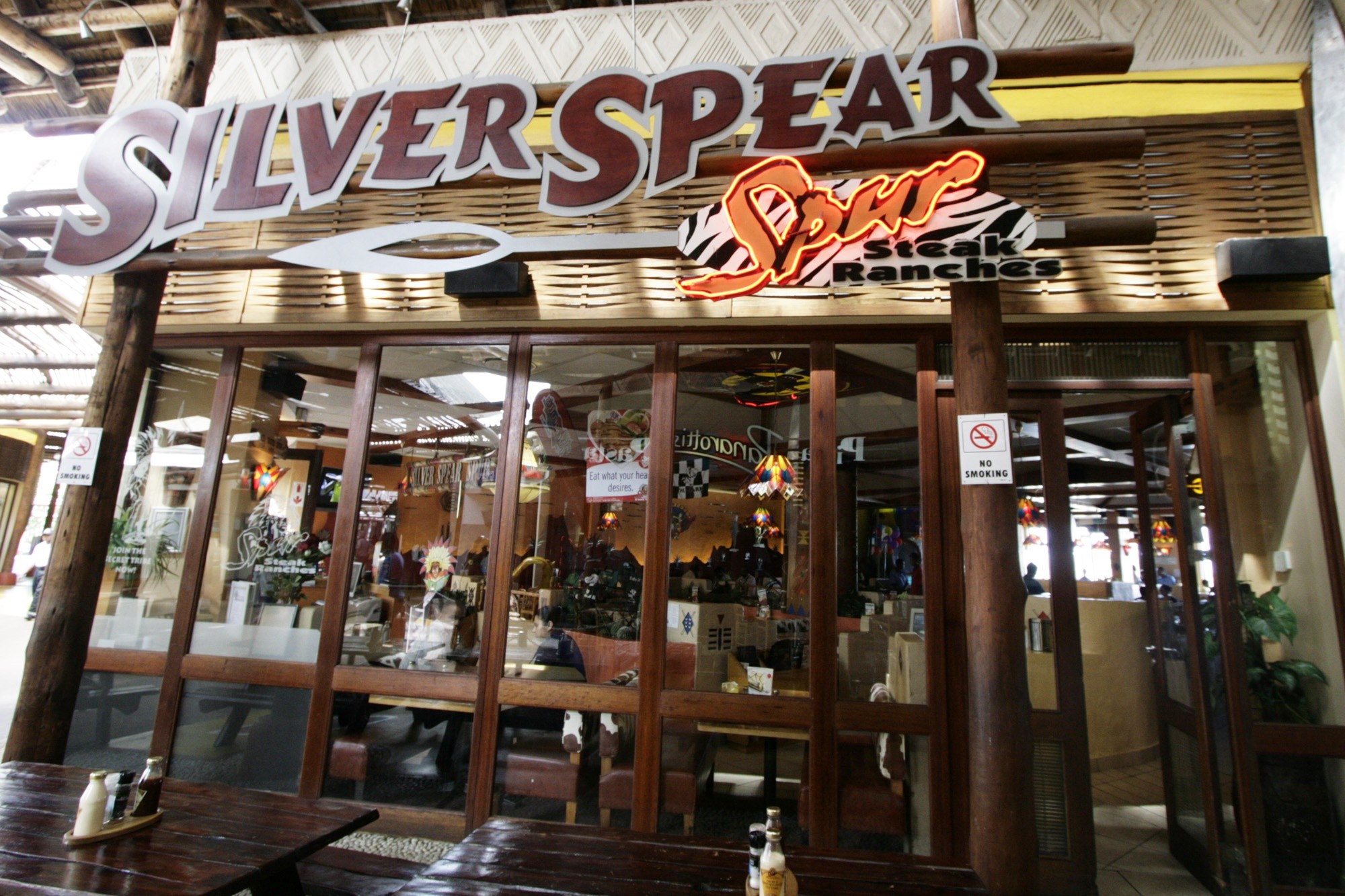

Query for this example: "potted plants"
[1199,584,1344,892]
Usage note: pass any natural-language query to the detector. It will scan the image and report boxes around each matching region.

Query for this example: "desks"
[694,670,810,743]
[369,654,519,715]
[0,760,378,896]
[388,814,989,896]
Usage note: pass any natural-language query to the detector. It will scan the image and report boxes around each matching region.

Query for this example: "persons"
[496,605,587,752]
[1079,570,1089,581]
[1156,567,1177,585]
[398,601,473,795]
[377,532,406,604]
[25,517,1179,795]
[25,527,54,614]
[1023,563,1046,595]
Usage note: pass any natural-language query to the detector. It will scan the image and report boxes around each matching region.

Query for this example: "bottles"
[456,634,463,653]
[132,756,163,817]
[102,772,121,829]
[760,827,785,896]
[352,622,360,636]
[382,621,392,644]
[766,806,782,830]
[748,831,767,889]
[73,770,108,836]
[749,823,766,833]
[112,770,136,820]
[407,661,417,670]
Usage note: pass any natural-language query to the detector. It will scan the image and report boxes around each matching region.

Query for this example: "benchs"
[494,619,717,840]
[797,683,913,834]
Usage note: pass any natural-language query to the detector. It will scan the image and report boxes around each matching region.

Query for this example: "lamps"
[78,0,162,98]
